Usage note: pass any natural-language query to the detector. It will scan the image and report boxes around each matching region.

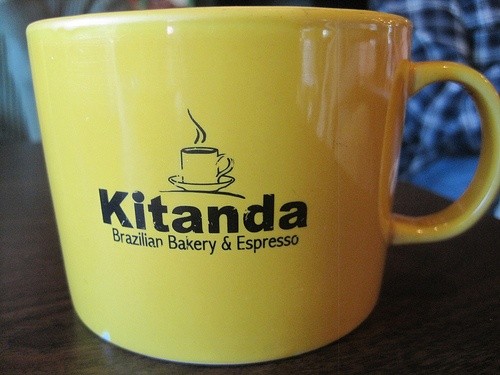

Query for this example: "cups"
[25,5,499,370]
